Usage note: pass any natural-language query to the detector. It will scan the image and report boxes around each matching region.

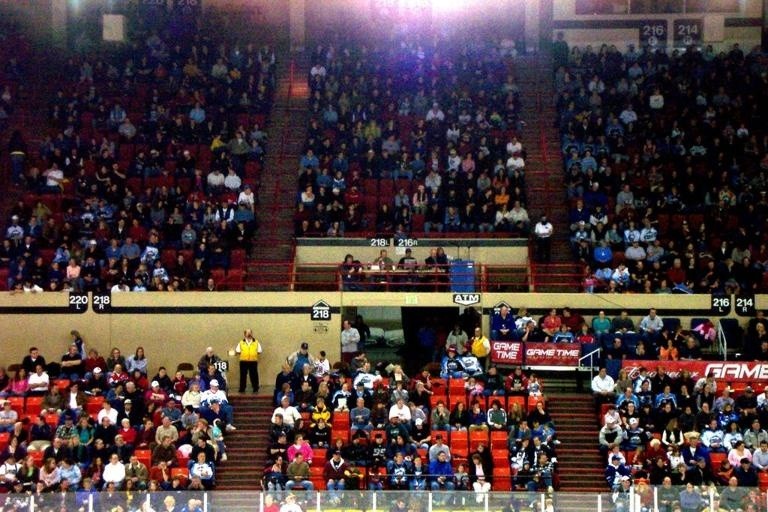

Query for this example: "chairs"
[0,81,768,492]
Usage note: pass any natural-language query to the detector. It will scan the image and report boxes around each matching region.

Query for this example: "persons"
[236,328,263,395]
[260,305,767,510]
[0,328,236,512]
[1,2,765,293]
